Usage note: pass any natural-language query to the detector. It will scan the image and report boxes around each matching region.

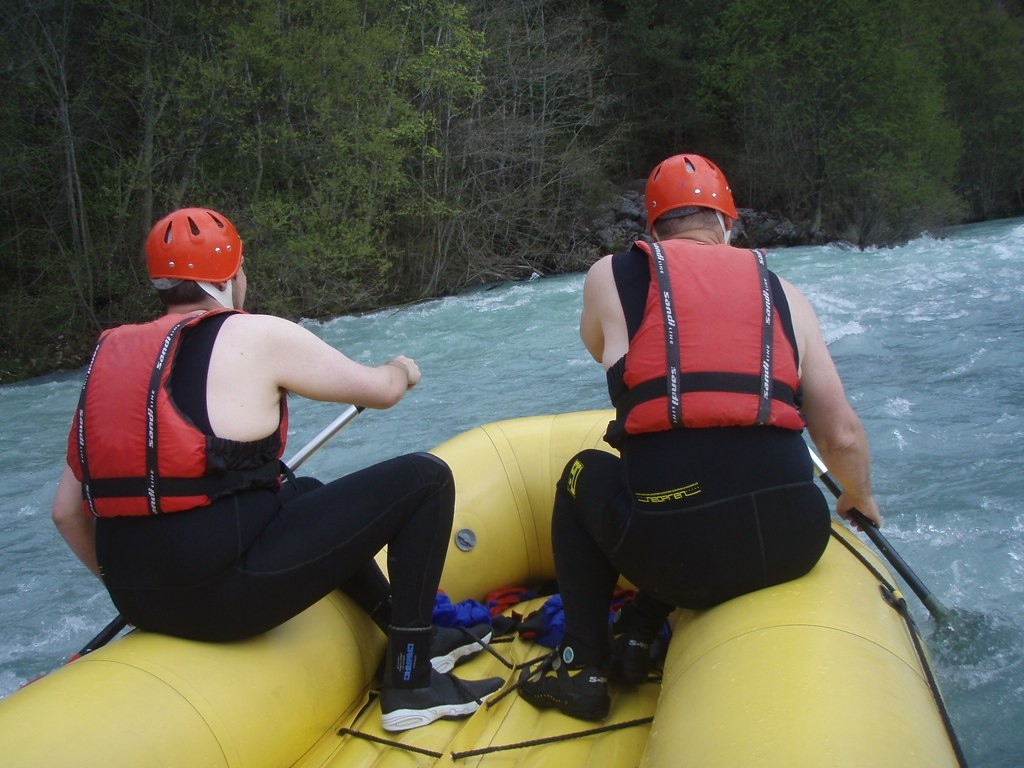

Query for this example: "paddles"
[16,403,367,689]
[805,444,948,620]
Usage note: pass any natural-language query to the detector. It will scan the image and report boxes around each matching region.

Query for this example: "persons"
[514,153,882,725]
[48,206,506,733]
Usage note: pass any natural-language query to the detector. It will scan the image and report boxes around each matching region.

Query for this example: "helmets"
[645,153,738,242]
[146,208,243,282]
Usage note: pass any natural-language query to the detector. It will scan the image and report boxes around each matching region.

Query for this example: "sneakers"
[518,645,610,720]
[606,605,651,683]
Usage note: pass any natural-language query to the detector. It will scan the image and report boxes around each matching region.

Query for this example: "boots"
[370,585,495,674]
[378,625,505,731]
